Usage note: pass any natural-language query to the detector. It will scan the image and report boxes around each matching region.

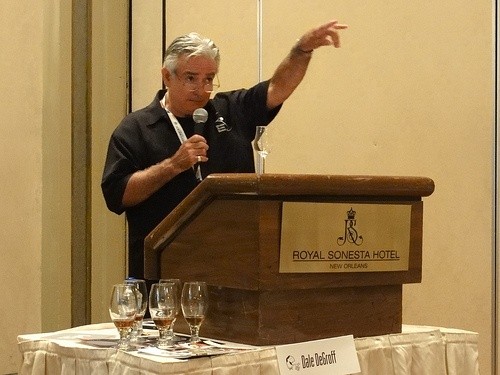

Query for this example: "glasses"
[173,69,221,93]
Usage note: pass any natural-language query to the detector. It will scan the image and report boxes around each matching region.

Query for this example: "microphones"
[193,108,208,166]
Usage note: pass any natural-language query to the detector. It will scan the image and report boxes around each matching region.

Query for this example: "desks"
[17,323,480,375]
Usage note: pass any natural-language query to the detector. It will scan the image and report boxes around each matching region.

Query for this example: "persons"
[100,21,348,321]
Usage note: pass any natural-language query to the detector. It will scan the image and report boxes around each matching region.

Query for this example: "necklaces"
[158,96,203,184]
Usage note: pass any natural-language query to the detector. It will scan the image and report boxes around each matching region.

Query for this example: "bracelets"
[291,47,314,54]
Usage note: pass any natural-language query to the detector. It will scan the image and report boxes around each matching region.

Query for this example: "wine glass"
[121,279,148,349]
[109,284,137,352]
[159,279,182,342]
[254,126,272,174]
[181,281,210,347]
[148,282,177,350]
